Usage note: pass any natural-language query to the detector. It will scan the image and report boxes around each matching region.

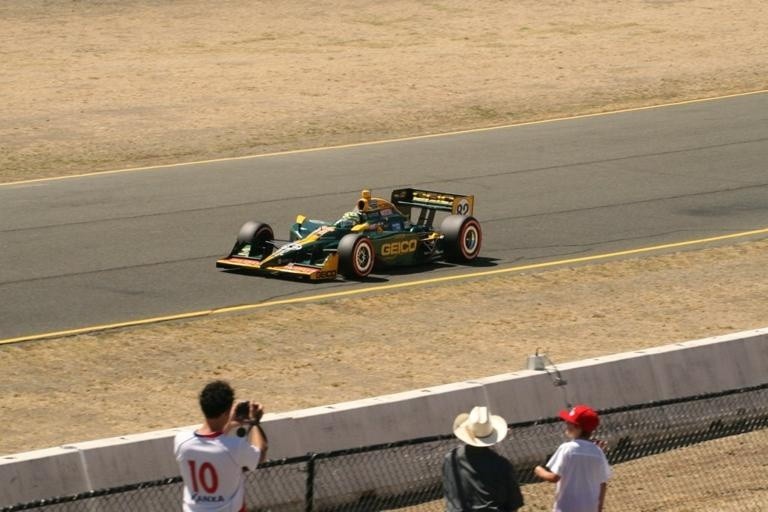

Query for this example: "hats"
[451,404,510,447]
[557,404,602,433]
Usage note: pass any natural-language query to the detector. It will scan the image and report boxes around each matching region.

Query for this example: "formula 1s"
[216,187,483,283]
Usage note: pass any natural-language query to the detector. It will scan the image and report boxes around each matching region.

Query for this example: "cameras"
[236,401,258,419]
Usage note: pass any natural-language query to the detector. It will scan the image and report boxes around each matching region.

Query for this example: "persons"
[172,380,267,512]
[439,404,525,512]
[533,406,609,512]
